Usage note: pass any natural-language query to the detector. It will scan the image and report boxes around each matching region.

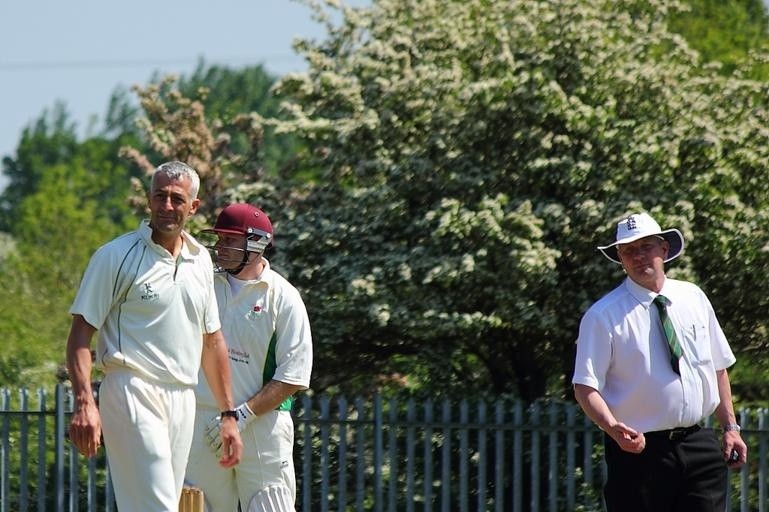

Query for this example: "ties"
[654,294,683,375]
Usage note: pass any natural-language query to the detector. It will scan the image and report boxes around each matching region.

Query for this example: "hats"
[598,213,684,263]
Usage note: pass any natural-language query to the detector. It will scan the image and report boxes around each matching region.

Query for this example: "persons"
[65,160,243,512]
[571,212,748,511]
[180,201,313,512]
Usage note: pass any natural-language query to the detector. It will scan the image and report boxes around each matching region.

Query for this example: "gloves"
[206,400,259,456]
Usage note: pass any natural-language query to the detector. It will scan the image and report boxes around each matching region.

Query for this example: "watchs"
[221,409,239,420]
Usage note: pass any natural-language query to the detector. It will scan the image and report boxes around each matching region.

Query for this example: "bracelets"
[725,424,740,432]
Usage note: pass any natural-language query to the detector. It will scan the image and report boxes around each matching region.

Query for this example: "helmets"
[201,204,273,254]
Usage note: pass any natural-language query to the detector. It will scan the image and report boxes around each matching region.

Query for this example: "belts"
[656,430,688,440]
